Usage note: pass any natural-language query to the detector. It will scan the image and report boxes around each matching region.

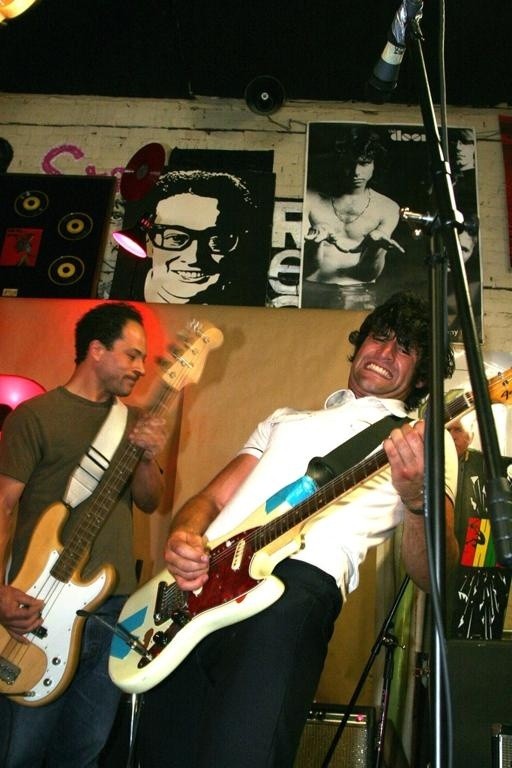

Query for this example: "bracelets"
[402,488,425,515]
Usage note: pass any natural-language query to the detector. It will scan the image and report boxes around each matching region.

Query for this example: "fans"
[246,73,307,135]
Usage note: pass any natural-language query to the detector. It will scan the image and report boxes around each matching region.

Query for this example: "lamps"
[112,211,156,259]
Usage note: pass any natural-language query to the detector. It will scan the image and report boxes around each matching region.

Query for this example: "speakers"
[430,638,512,768]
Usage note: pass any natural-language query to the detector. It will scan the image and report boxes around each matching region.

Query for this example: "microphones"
[364,0,423,105]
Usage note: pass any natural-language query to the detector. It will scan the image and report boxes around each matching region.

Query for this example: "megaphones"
[243,74,288,116]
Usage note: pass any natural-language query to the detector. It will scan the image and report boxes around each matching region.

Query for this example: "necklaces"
[332,186,372,224]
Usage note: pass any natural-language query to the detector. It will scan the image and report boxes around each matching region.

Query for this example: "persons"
[112,170,264,307]
[129,293,460,768]
[303,128,406,309]
[448,418,483,537]
[443,218,480,330]
[434,128,477,215]
[0,304,171,768]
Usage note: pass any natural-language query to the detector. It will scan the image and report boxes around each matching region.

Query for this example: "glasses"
[140,215,248,257]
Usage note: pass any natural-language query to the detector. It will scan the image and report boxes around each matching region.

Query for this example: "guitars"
[108,367,512,694]
[0,318,224,708]
